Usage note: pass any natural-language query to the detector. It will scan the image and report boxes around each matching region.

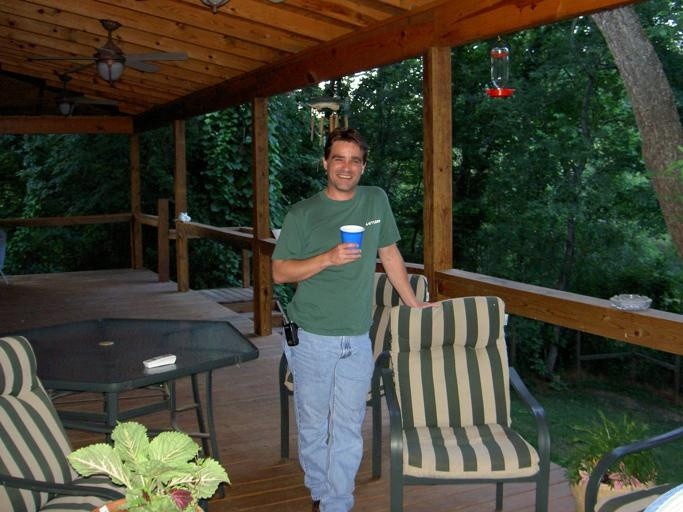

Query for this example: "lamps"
[58,103,75,115]
[97,59,125,84]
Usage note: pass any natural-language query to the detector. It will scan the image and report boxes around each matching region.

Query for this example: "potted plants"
[564,411,658,512]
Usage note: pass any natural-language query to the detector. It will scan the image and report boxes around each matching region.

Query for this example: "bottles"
[489,47,511,90]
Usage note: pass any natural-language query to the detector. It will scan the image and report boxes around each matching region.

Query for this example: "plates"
[485,88,516,97]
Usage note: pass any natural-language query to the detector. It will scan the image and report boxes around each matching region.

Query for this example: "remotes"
[142,353,176,368]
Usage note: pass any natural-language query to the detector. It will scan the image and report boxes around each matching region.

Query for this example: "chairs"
[280,270,551,511]
[584,428,682,511]
[0,334,131,512]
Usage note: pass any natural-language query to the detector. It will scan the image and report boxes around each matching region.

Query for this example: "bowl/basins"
[611,293,654,313]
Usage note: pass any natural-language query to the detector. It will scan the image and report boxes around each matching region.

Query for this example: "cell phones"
[284,322,299,346]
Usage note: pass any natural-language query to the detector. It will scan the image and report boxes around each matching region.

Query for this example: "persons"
[270,125,441,511]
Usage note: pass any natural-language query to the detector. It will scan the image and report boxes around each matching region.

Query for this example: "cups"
[339,225,365,255]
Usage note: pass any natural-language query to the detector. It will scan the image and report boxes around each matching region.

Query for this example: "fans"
[24,75,120,114]
[31,19,187,74]
